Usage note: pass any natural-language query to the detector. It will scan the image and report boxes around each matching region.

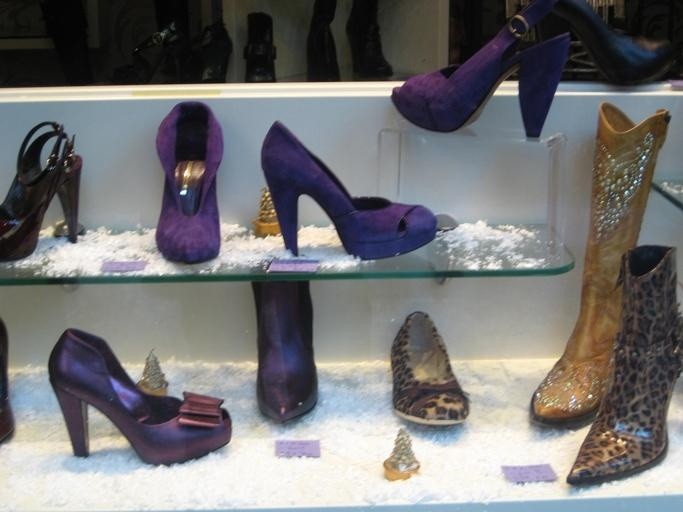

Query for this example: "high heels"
[111,21,178,85]
[391,0,572,138]
[532,0,676,86]
[0,120,83,263]
[261,121,437,260]
[48,328,232,465]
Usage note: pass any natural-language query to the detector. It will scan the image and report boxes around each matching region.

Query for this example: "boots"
[528,102,671,431]
[195,21,233,83]
[306,0,340,82]
[244,11,277,82]
[251,278,319,423]
[565,245,682,488]
[345,0,394,78]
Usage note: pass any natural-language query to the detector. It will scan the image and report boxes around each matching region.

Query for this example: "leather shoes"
[386,311,470,426]
[155,100,224,265]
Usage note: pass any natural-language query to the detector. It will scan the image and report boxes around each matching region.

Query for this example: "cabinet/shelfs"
[3,78,679,512]
